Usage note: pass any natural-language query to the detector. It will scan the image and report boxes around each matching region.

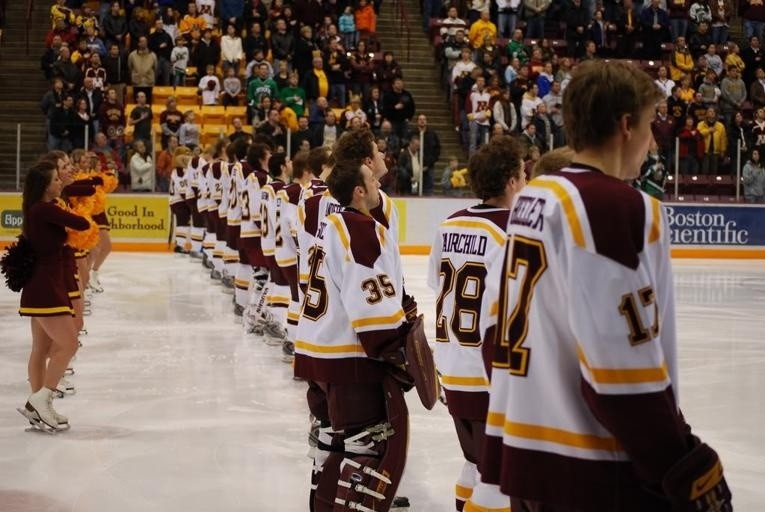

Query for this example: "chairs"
[120,82,346,164]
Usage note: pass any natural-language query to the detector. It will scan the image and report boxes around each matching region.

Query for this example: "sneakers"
[54,376,72,390]
[29,392,69,425]
[26,388,57,428]
[174,246,304,380]
[65,269,103,375]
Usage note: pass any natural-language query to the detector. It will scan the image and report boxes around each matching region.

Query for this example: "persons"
[480,54,732,512]
[44,1,765,204]
[425,131,530,512]
[166,123,423,461]
[289,156,420,512]
[1,130,118,434]
